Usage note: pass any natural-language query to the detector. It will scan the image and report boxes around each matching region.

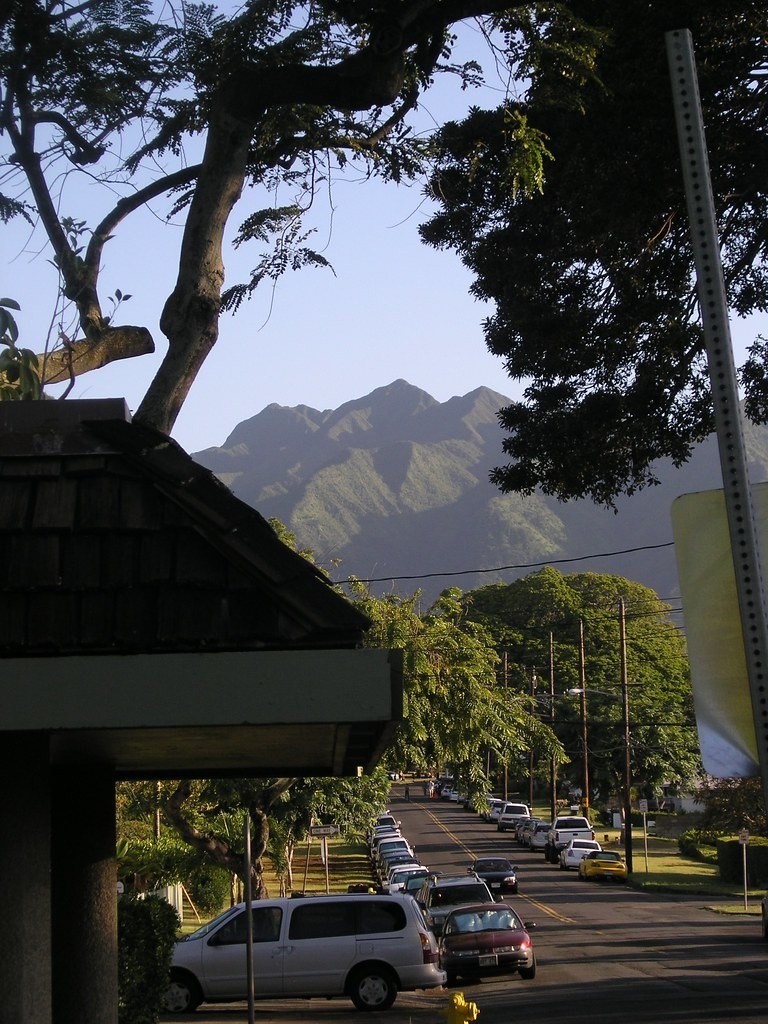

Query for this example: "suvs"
[148,891,449,1014]
[497,802,533,833]
[415,870,504,944]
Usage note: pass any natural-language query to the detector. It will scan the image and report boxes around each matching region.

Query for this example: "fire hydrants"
[437,991,480,1024]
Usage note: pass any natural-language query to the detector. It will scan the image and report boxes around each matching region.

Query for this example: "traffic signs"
[309,823,341,839]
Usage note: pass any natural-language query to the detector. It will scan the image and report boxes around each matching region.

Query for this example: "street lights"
[569,688,632,873]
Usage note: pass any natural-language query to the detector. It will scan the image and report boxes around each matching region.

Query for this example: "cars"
[436,903,538,985]
[578,850,629,883]
[558,838,603,870]
[466,856,520,898]
[365,774,552,897]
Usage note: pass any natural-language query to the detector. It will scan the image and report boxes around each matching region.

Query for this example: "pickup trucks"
[544,816,595,864]
